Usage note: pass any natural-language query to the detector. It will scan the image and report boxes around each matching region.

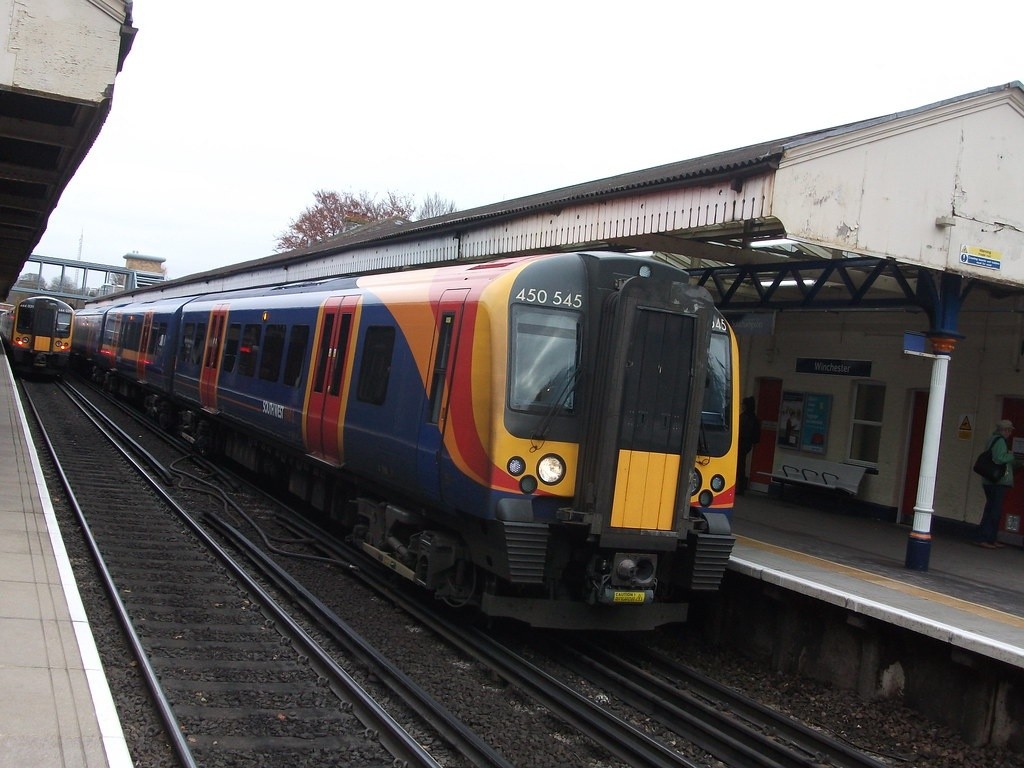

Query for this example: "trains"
[0,296,74,376]
[72,250,740,629]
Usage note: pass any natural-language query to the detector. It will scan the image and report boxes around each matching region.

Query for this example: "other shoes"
[994,543,1007,547]
[735,488,745,496]
[975,542,996,548]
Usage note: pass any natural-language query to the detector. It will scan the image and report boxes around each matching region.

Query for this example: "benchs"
[756,454,867,514]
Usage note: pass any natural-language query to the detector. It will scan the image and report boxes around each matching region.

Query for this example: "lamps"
[760,277,815,287]
[747,237,800,248]
[626,248,654,257]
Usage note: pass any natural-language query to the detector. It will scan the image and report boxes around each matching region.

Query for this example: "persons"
[978,420,1014,549]
[736,396,761,494]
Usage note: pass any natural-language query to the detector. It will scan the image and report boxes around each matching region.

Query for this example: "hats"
[1000,420,1015,429]
[740,396,755,406]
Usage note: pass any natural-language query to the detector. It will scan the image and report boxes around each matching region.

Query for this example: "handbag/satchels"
[973,435,1009,482]
